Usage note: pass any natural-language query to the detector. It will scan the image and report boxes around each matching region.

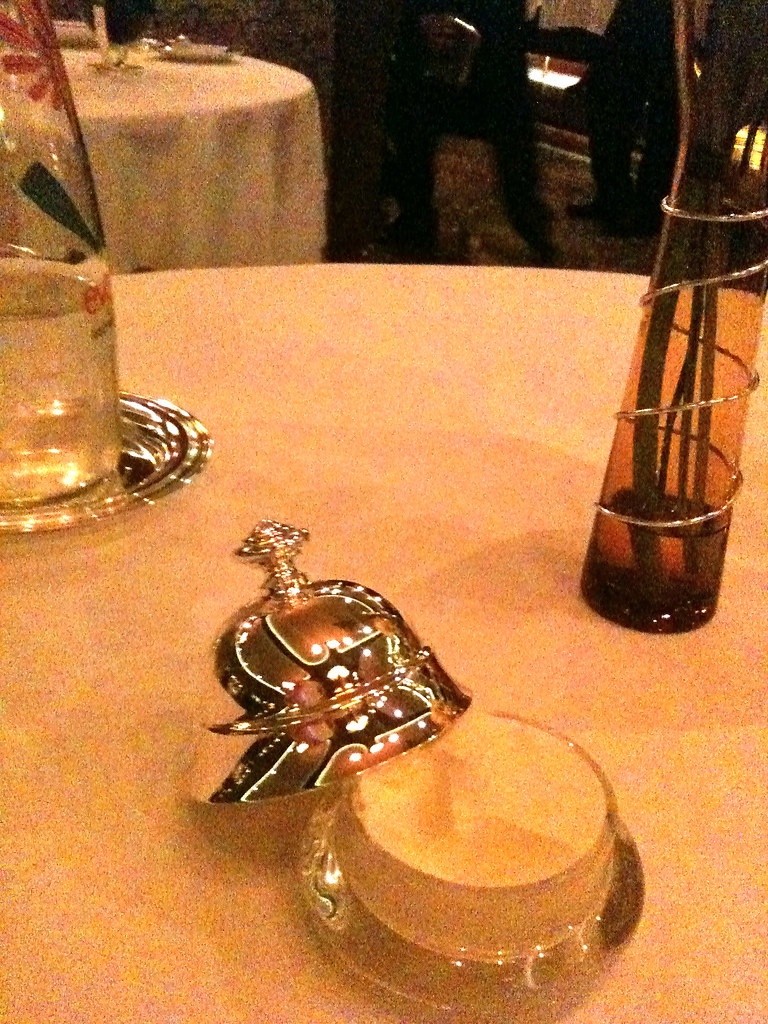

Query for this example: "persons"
[355,0,561,268]
[567,1,685,238]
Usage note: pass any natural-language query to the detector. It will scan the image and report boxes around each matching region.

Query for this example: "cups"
[329,711,621,967]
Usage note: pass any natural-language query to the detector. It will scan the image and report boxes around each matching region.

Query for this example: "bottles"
[1,3,126,503]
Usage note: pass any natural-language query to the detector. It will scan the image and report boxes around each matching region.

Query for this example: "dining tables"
[0,255,768,1024]
[0,41,334,265]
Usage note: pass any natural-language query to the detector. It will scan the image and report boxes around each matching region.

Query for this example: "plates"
[300,775,645,1023]
[1,388,219,542]
